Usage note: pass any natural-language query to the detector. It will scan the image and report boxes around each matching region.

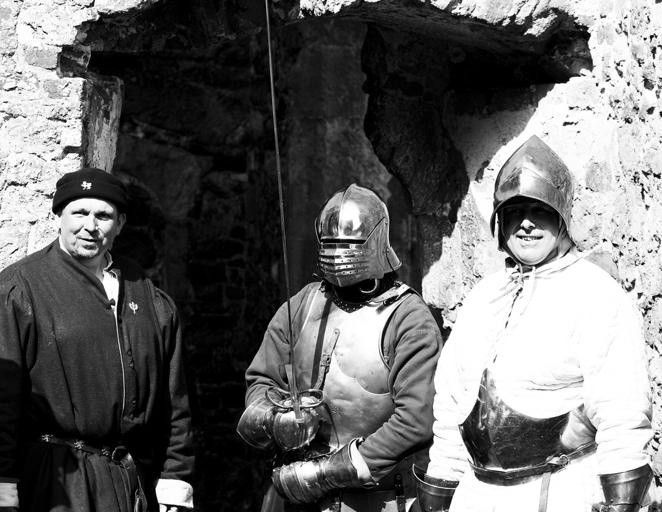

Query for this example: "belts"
[41,432,143,512]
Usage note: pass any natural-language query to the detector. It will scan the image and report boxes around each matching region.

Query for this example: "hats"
[52,167,130,215]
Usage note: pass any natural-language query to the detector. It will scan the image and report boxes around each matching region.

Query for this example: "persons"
[0,168,201,511]
[409,134,656,512]
[234,181,443,511]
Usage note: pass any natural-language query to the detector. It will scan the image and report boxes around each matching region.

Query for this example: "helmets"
[490,134,576,247]
[313,182,402,288]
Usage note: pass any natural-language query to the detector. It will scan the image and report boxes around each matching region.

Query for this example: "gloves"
[271,436,380,508]
[236,397,332,449]
